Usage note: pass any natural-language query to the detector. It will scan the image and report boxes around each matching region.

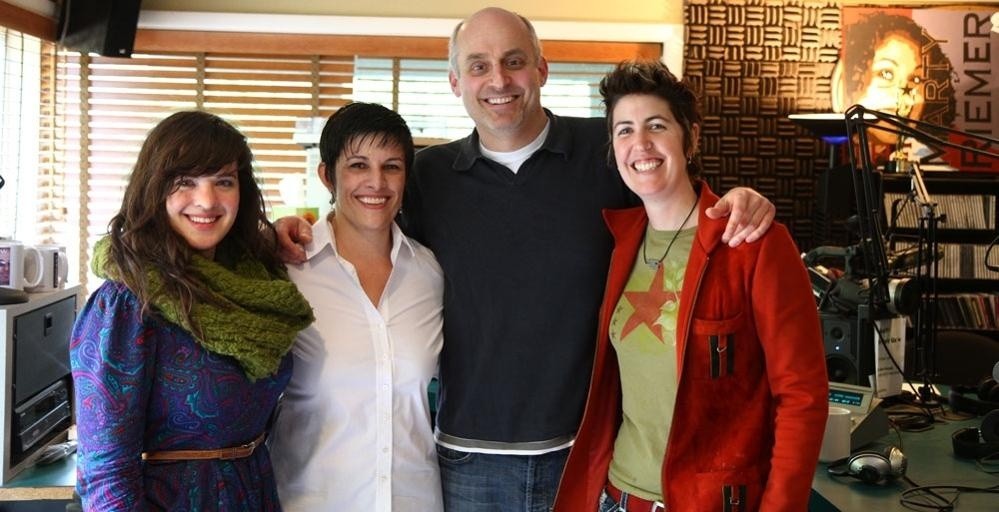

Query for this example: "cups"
[818,407,851,462]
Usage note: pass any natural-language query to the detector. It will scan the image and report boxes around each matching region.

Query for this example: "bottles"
[0,241,68,293]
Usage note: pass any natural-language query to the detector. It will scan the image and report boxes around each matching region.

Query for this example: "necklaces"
[641,198,699,271]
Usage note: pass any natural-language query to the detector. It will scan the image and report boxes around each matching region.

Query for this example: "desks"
[808,384,999,511]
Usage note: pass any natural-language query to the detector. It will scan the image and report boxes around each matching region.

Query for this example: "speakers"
[817,310,874,386]
[56,0,141,58]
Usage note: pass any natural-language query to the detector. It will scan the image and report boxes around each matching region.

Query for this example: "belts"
[143,433,266,461]
[605,483,665,512]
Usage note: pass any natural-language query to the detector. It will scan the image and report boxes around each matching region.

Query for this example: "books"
[880,192,999,337]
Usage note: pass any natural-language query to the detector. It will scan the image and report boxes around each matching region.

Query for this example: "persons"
[68,110,319,512]
[831,15,958,173]
[271,100,451,508]
[262,4,777,508]
[548,56,828,510]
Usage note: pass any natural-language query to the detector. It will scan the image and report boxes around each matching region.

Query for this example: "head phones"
[949,376,999,416]
[952,410,999,459]
[827,445,907,481]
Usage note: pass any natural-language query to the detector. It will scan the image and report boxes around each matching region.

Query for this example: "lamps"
[787,113,881,244]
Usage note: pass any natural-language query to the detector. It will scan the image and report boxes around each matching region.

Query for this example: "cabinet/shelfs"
[875,167,995,336]
